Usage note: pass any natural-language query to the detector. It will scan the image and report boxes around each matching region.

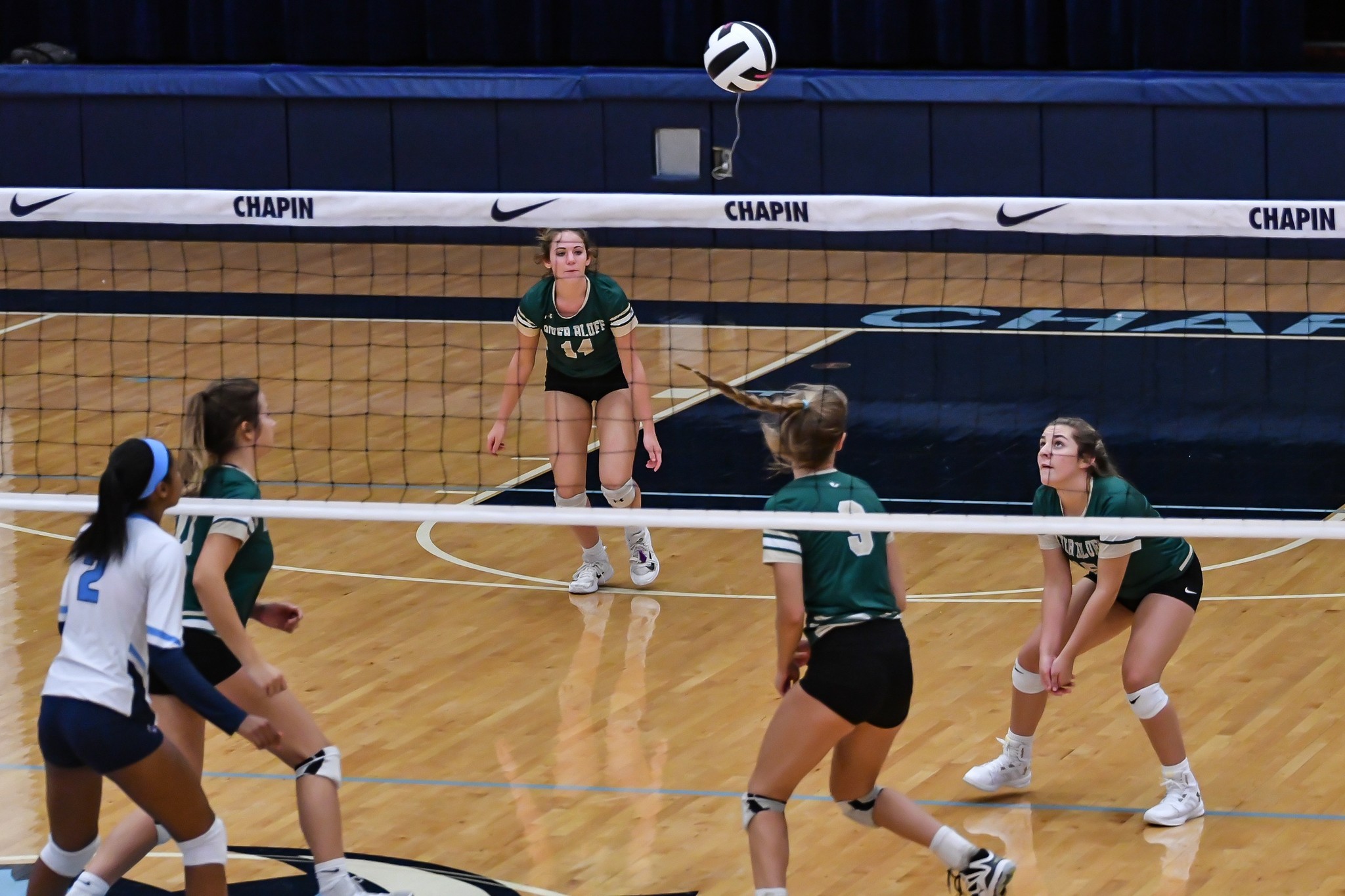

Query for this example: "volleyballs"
[703,21,776,93]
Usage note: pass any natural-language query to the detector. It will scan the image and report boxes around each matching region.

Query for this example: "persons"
[963,417,1204,826]
[487,229,662,593]
[26,438,282,896]
[64,378,414,896]
[674,361,1016,896]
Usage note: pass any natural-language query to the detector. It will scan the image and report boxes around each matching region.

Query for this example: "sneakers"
[568,544,614,593]
[626,595,661,642]
[963,801,1032,843]
[1143,770,1205,826]
[1143,815,1205,854]
[625,526,660,586]
[316,872,413,896]
[947,847,1016,896]
[569,591,614,622]
[962,733,1031,792]
